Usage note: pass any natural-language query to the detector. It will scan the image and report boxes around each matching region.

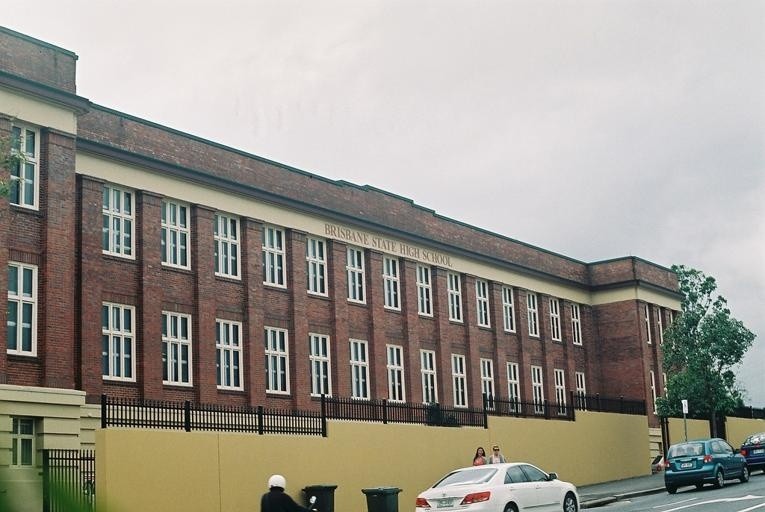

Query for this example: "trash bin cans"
[361,487,403,512]
[301,485,338,512]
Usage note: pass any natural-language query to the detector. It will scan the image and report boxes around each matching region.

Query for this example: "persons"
[260,474,314,512]
[487,445,506,464]
[472,447,488,465]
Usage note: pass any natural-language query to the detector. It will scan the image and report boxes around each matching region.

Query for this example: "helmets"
[268,475,286,491]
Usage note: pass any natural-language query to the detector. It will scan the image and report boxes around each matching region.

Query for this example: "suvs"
[665,437,750,495]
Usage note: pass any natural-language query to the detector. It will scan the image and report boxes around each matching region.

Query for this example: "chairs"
[676,447,684,455]
[699,447,702,455]
[686,447,694,455]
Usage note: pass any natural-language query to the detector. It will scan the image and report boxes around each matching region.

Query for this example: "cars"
[416,463,580,512]
[652,455,665,473]
[740,433,765,474]
